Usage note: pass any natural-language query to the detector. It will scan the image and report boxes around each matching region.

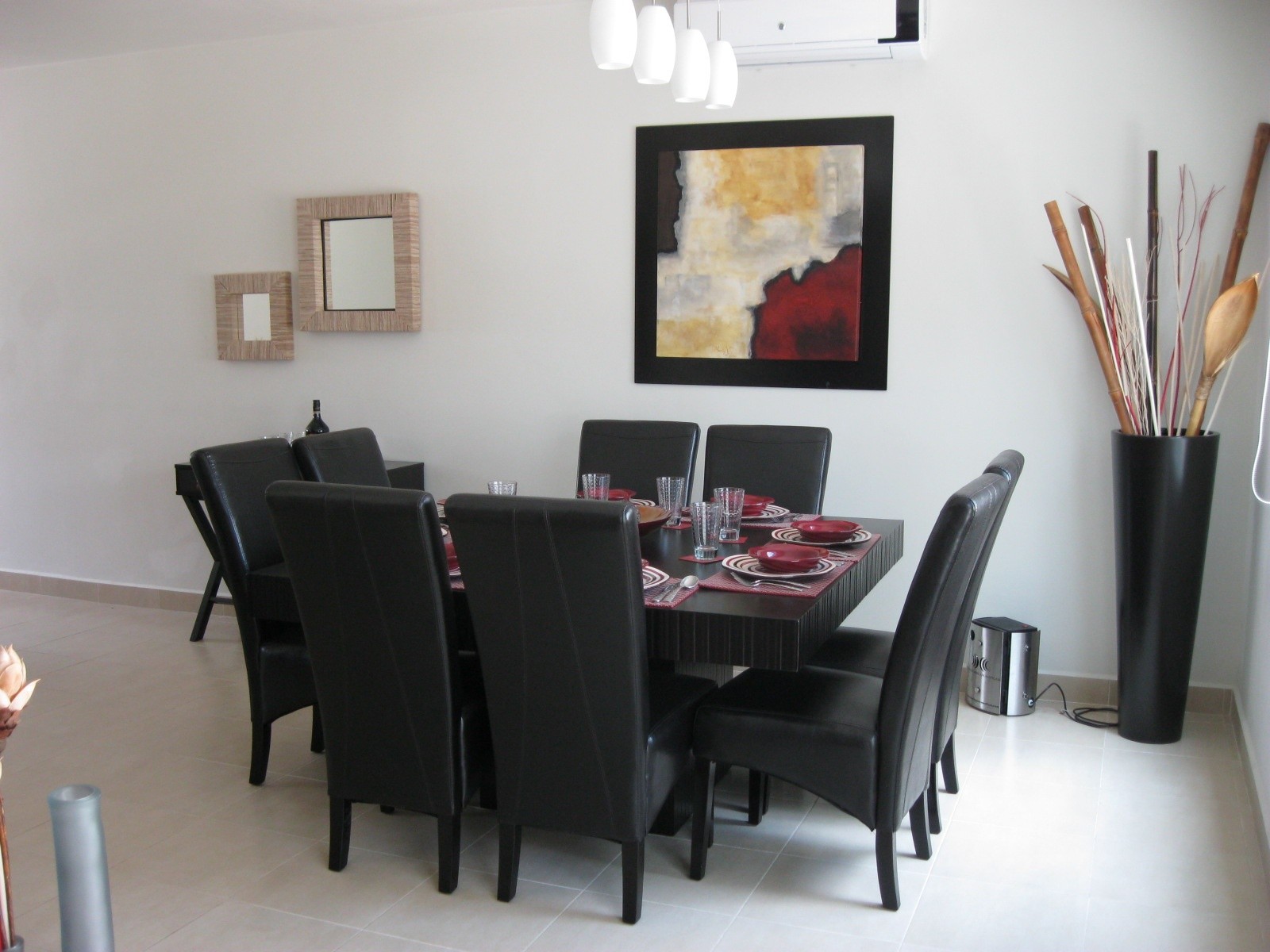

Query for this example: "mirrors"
[296,193,421,332]
[212,272,295,362]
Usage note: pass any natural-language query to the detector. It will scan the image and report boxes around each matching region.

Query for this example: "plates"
[450,571,461,575]
[577,488,637,499]
[643,566,669,589]
[722,554,837,579]
[630,498,657,506]
[772,527,872,546]
[740,505,794,521]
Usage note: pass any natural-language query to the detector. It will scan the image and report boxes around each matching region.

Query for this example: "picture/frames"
[633,116,894,391]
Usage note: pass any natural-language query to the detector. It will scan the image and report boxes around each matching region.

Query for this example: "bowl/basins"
[791,521,864,541]
[641,558,649,569]
[710,494,777,515]
[636,506,676,536]
[747,544,831,571]
[444,542,460,571]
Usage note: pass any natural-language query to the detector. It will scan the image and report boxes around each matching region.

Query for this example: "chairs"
[266,480,497,895]
[762,448,1025,833]
[702,425,832,514]
[689,473,1010,911]
[191,436,396,813]
[575,418,702,506]
[291,426,391,488]
[443,492,719,923]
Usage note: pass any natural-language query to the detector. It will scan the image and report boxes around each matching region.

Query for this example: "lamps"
[631,0,676,85]
[702,0,739,110]
[670,0,711,103]
[588,0,637,69]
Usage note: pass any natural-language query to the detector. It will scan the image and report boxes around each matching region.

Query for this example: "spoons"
[664,575,700,602]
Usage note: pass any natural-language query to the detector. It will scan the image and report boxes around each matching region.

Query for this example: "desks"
[176,461,426,641]
[436,507,903,837]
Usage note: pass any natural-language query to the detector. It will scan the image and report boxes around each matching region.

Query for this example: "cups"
[690,502,723,559]
[263,430,305,444]
[656,476,687,526]
[487,481,517,496]
[714,487,745,541]
[582,473,610,502]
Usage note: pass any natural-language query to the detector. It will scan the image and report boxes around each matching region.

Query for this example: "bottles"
[305,400,330,435]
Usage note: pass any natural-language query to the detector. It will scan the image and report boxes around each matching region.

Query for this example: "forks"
[730,571,813,591]
